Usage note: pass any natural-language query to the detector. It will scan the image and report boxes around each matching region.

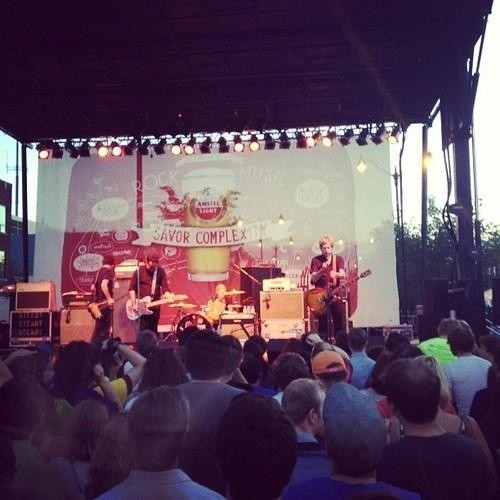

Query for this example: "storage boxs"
[261,321,307,345]
[14,281,57,312]
[9,310,60,350]
[260,290,306,321]
[62,307,105,344]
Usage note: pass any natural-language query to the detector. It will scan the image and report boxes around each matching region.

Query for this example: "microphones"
[136,260,139,271]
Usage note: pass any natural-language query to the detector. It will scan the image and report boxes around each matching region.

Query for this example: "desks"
[214,313,258,343]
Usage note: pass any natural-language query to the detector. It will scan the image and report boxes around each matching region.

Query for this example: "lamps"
[36,126,404,162]
[232,213,302,264]
[355,154,399,178]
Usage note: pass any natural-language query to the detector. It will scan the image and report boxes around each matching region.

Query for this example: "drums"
[227,304,243,313]
[177,312,216,338]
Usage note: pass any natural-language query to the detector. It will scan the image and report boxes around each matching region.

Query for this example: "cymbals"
[169,302,197,309]
[223,290,246,296]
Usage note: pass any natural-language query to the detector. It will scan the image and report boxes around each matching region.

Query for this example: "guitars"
[89,293,130,319]
[305,268,371,314]
[126,293,188,320]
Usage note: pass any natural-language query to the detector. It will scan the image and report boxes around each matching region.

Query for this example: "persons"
[309,237,348,347]
[90,255,118,349]
[1,324,499,499]
[128,251,173,331]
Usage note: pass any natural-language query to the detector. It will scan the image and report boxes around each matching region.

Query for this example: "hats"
[312,350,346,374]
[305,333,324,346]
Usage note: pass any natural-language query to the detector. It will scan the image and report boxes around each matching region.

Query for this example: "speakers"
[455,279,484,341]
[60,309,96,345]
[113,278,139,344]
[366,335,387,354]
[267,339,311,364]
[416,277,449,342]
